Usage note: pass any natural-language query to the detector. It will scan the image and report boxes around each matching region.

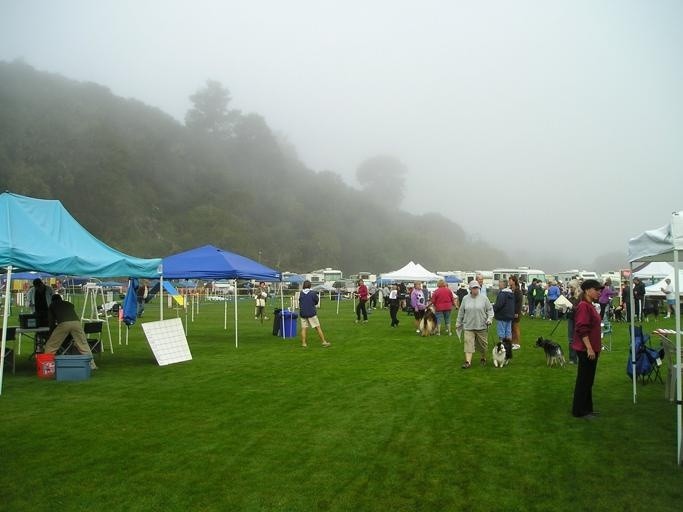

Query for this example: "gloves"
[486,318,492,325]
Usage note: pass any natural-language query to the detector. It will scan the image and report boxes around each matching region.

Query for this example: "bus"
[492,267,547,293]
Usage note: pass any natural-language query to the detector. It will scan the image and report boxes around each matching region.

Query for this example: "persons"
[355,279,378,323]
[44,294,98,370]
[567,280,604,420]
[299,281,331,348]
[456,276,522,368]
[255,282,269,319]
[136,278,150,318]
[383,279,455,336]
[519,276,675,319]
[26,278,55,327]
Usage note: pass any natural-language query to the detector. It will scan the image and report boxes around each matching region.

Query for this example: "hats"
[469,281,480,289]
[582,280,605,291]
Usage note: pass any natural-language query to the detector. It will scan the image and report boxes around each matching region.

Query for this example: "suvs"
[545,274,562,286]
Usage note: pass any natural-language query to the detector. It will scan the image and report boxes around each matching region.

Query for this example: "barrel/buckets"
[35,353,56,377]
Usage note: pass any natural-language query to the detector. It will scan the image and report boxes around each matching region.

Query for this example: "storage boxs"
[55,355,91,380]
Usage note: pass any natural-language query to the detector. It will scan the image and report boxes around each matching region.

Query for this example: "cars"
[61,278,259,297]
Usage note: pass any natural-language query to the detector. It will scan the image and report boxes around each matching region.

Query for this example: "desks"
[10,325,51,358]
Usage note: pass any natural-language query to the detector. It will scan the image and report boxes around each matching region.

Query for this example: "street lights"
[258,248,264,263]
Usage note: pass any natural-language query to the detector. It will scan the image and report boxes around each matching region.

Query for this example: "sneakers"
[321,343,331,347]
[511,344,521,350]
[462,361,471,368]
[480,357,487,367]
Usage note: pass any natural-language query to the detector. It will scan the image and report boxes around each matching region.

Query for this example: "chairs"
[0,326,16,375]
[630,325,665,383]
[600,322,611,350]
[81,321,102,354]
[659,334,683,401]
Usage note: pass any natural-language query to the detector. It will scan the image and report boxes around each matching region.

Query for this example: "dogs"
[492,337,514,368]
[533,336,567,368]
[419,307,437,337]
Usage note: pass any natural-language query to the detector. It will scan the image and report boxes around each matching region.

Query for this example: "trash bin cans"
[279,310,298,338]
[272,310,282,336]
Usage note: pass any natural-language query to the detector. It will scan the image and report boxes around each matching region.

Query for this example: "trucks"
[251,267,492,296]
[600,271,620,292]
[558,270,599,288]
